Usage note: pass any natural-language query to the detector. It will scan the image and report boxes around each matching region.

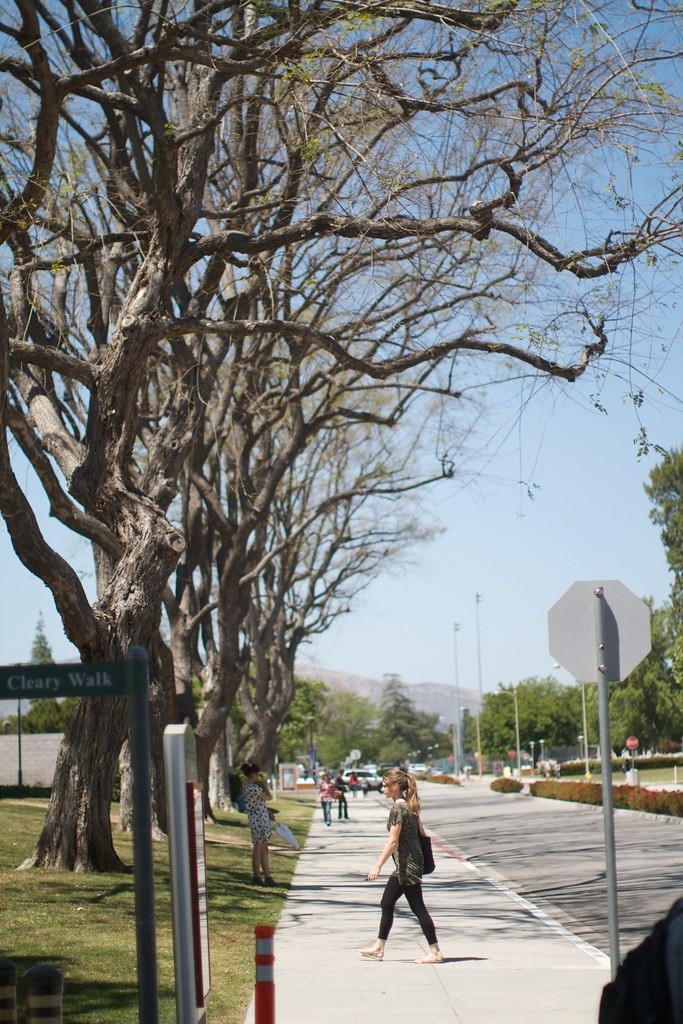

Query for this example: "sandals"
[415,951,444,963]
[357,949,384,961]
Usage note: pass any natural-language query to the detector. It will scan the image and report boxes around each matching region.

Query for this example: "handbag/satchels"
[415,821,435,875]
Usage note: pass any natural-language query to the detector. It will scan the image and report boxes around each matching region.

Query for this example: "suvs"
[338,768,386,793]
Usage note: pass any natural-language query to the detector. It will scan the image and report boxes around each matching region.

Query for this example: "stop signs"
[627,737,639,749]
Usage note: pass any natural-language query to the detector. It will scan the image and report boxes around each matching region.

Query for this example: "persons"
[622,759,632,786]
[359,770,444,962]
[240,762,278,887]
[316,767,369,826]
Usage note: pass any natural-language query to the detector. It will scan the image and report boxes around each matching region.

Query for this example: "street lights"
[474,591,484,777]
[553,662,592,778]
[494,690,521,778]
[530,741,535,777]
[539,739,545,776]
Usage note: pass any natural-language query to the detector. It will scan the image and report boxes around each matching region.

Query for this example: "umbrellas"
[273,820,300,850]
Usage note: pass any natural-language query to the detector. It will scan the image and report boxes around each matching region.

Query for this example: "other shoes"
[265,876,279,886]
[327,821,330,826]
[251,877,267,887]
